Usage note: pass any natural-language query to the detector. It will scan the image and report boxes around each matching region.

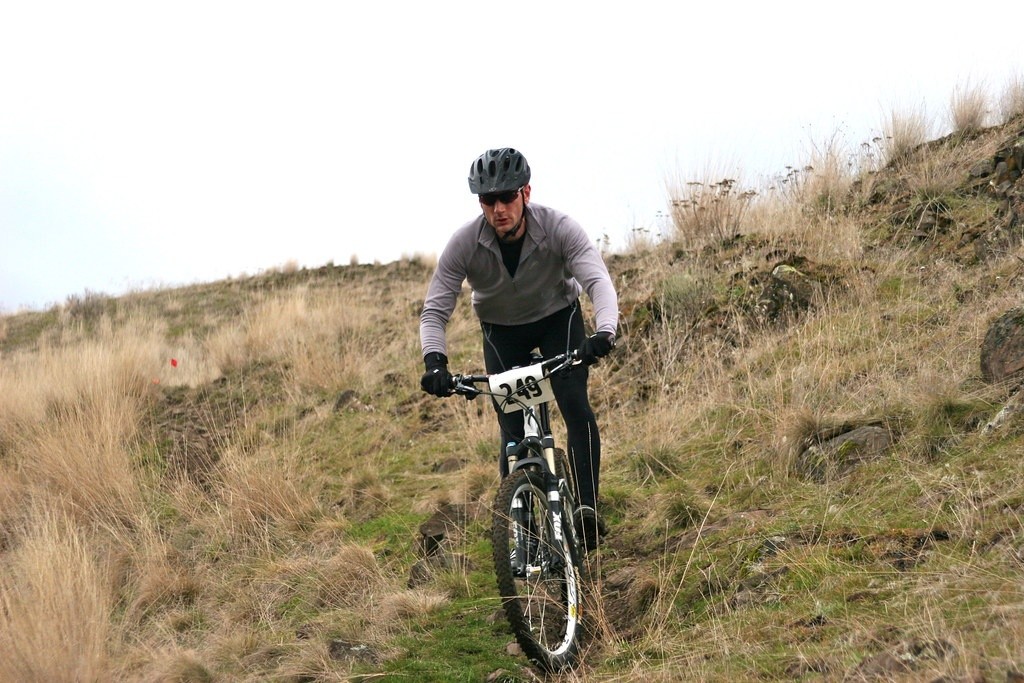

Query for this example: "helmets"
[469,149,531,194]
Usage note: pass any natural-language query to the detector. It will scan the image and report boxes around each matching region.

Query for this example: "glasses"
[476,185,525,206]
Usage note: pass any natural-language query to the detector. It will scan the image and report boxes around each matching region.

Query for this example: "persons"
[419,148,617,578]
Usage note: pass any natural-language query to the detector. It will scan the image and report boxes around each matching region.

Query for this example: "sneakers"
[509,538,538,577]
[573,506,604,549]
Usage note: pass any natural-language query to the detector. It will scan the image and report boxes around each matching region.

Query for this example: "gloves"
[577,330,616,365]
[419,357,455,397]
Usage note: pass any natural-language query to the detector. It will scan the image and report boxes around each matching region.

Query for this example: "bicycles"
[419,340,618,671]
